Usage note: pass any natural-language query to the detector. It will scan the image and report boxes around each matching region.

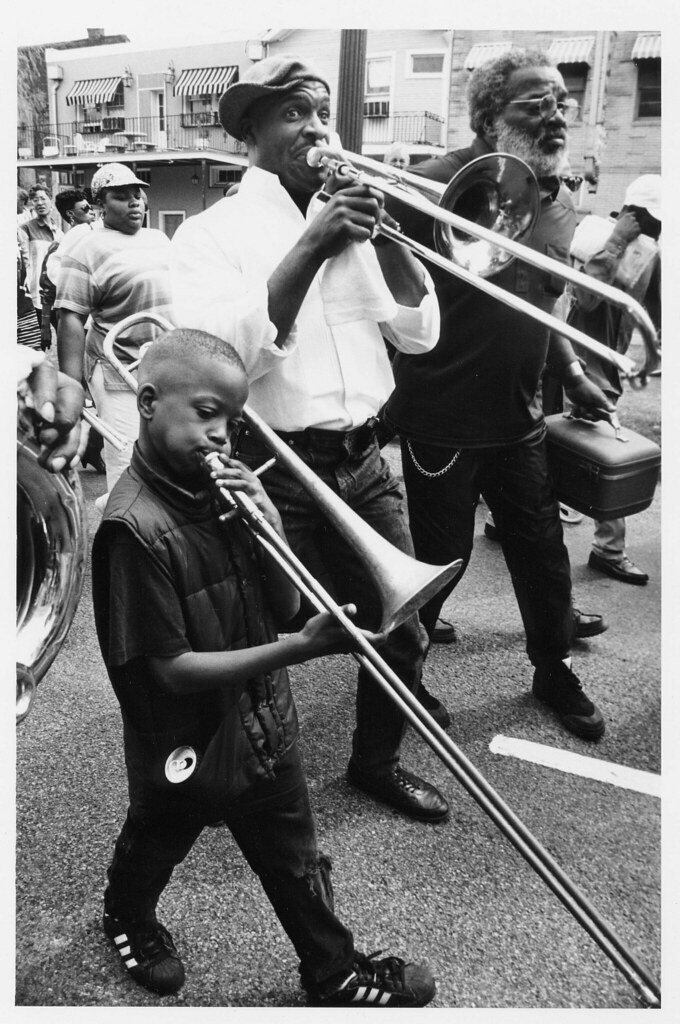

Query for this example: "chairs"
[42,133,109,158]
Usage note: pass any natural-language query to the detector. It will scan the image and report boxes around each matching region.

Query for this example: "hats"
[218,53,330,142]
[91,163,150,203]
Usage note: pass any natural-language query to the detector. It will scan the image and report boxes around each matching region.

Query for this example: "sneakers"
[308,948,435,1007]
[346,762,449,822]
[408,670,450,729]
[533,660,605,737]
[103,907,185,995]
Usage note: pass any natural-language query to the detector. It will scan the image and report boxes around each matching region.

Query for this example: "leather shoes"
[484,523,500,543]
[588,551,649,585]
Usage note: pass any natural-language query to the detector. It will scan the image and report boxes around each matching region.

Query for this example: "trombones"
[101,310,661,1008]
[305,139,658,378]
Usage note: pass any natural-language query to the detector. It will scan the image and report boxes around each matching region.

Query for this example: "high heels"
[80,448,106,474]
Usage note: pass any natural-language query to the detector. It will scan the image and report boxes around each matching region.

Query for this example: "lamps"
[166,68,175,83]
[190,170,199,184]
[122,71,133,87]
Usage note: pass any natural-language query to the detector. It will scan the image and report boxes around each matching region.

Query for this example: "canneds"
[164,745,204,783]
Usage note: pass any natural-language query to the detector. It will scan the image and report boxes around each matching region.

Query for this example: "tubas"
[17,390,88,725]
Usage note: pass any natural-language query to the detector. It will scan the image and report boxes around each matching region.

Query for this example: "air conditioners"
[102,116,120,131]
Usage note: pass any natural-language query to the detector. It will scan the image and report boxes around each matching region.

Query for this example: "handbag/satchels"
[569,214,658,365]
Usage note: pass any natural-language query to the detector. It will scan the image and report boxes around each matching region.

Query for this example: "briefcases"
[540,404,661,522]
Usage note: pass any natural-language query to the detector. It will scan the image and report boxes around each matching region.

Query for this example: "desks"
[63,145,77,157]
[114,132,147,153]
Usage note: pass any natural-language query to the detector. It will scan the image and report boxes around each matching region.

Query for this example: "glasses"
[560,175,582,192]
[500,94,581,123]
[69,205,93,213]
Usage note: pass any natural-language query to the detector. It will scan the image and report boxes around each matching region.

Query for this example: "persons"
[18,162,170,512]
[382,48,604,740]
[382,140,410,170]
[165,54,449,822]
[92,328,436,1007]
[570,170,662,583]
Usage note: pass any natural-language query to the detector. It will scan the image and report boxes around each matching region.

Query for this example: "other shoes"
[558,501,584,523]
[430,617,456,642]
[571,597,608,638]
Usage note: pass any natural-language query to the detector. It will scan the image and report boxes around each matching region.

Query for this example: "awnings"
[632,32,661,62]
[173,66,237,97]
[547,38,595,67]
[463,44,511,73]
[66,78,122,106]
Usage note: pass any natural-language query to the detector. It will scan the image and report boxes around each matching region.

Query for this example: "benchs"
[105,145,128,153]
[133,142,157,152]
